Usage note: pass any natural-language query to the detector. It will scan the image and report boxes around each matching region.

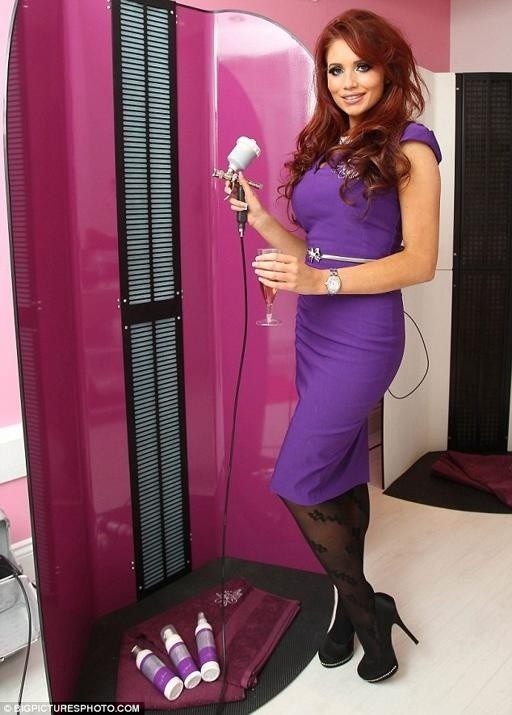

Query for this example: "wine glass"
[253,248,284,327]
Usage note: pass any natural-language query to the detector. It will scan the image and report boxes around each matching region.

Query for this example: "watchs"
[324,267,342,296]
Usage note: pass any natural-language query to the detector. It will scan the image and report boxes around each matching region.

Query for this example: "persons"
[224,9,442,683]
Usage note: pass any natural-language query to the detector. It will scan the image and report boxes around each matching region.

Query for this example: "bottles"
[160,624,202,689]
[130,644,183,701]
[194,612,221,682]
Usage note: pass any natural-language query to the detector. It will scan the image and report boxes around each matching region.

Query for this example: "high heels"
[318,581,375,668]
[358,592,418,683]
